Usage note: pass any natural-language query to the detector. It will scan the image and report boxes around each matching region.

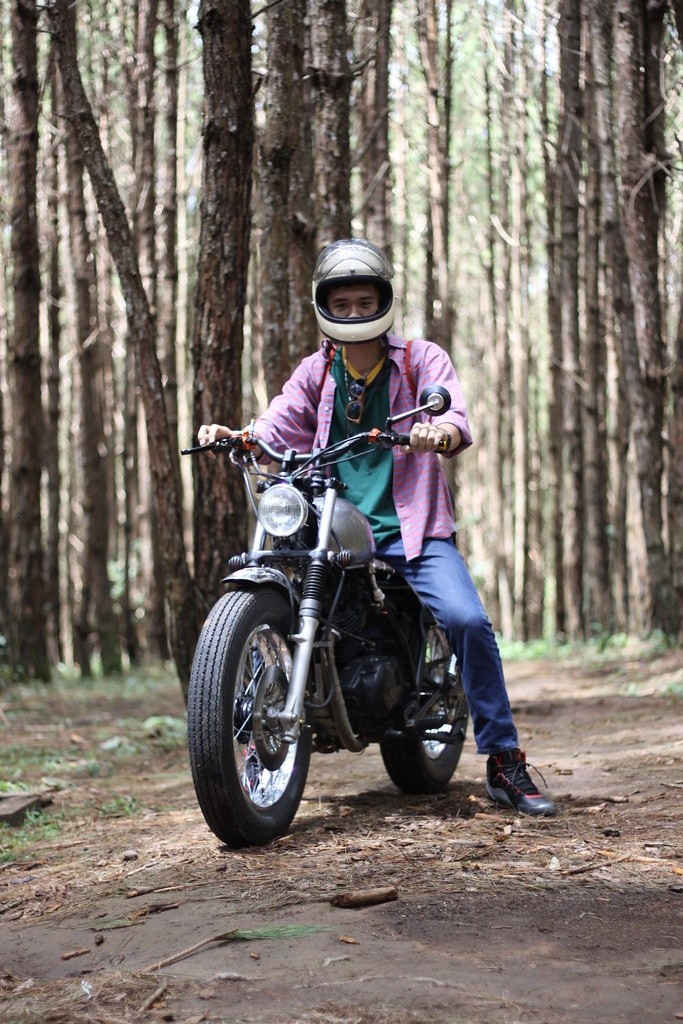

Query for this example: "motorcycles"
[177,383,470,852]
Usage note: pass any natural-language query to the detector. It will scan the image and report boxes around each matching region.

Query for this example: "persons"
[193,235,558,812]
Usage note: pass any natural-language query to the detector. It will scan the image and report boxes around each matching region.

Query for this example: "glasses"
[345,375,366,424]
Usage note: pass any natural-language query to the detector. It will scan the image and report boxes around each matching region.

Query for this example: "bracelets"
[436,431,452,455]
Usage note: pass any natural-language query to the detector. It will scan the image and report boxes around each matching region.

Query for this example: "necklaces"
[340,344,384,440]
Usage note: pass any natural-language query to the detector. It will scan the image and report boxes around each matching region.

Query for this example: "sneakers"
[485,747,557,817]
[242,745,263,791]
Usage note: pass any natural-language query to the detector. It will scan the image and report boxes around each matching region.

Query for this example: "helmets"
[311,237,395,344]
